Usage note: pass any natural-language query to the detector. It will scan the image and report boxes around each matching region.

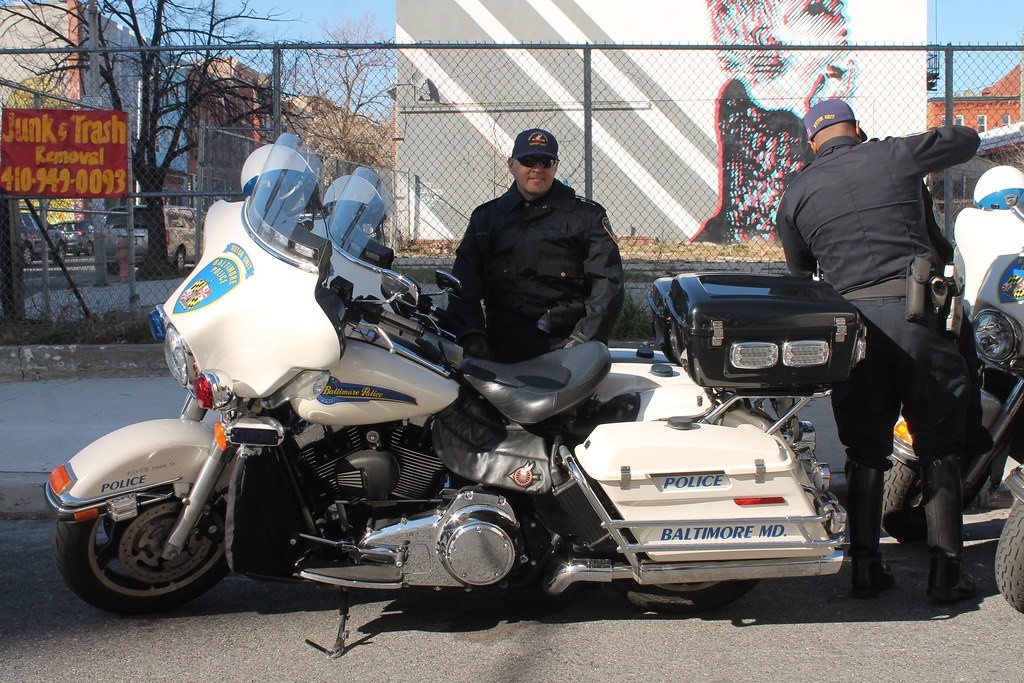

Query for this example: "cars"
[50,220,97,257]
[99,204,208,276]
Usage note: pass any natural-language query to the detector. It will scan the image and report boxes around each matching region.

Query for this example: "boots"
[844,456,895,599]
[924,465,976,602]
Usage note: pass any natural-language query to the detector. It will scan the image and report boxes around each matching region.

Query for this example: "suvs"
[18,212,68,265]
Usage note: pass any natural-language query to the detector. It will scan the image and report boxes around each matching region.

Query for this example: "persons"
[448,130,625,360]
[777,99,983,602]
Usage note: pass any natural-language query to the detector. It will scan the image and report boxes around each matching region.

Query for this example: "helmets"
[240,143,319,199]
[321,174,383,222]
[972,167,1024,210]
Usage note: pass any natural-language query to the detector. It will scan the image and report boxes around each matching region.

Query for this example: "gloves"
[462,334,491,361]
[549,338,582,349]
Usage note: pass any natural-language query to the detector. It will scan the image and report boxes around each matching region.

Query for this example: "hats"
[511,129,560,162]
[802,100,867,142]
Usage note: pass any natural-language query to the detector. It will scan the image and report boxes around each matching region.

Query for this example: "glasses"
[518,155,555,169]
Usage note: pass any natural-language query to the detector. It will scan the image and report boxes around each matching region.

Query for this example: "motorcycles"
[880,165,1024,542]
[996,461,1024,616]
[37,131,870,660]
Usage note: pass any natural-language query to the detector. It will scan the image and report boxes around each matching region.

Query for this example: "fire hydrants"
[117,234,129,284]
